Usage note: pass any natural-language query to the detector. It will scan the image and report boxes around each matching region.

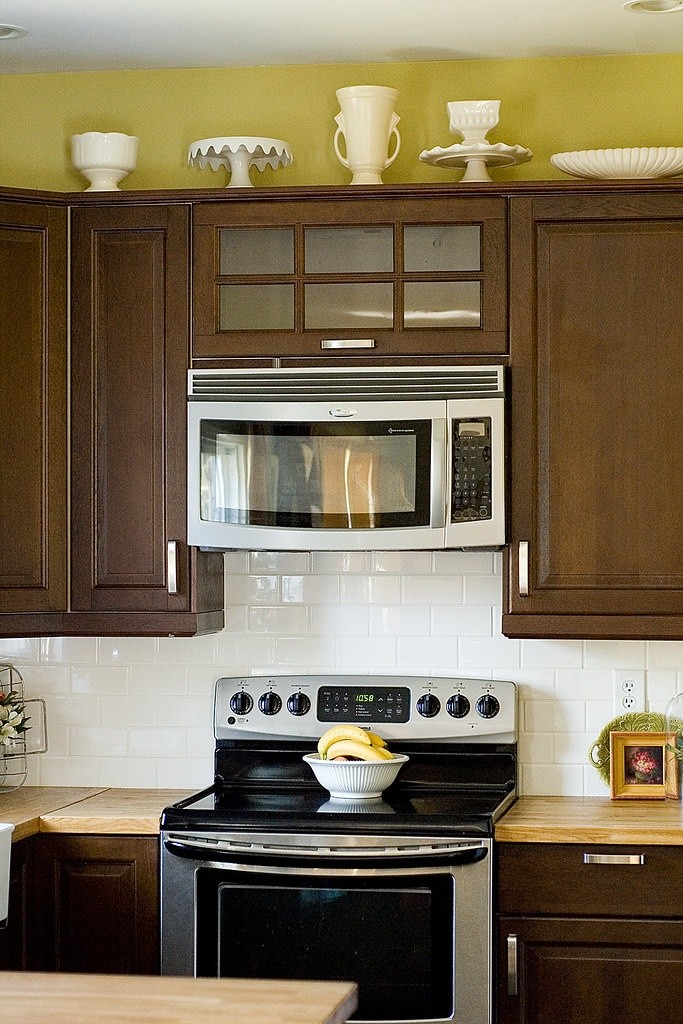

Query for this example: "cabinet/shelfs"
[0,178,683,642]
[0,832,161,976]
[495,839,683,1024]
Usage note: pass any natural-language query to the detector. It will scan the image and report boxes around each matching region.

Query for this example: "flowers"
[0,689,33,747]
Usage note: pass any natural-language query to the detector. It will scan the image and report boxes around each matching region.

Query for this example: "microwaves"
[184,364,509,552]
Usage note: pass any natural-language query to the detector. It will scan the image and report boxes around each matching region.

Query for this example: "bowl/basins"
[303,754,410,799]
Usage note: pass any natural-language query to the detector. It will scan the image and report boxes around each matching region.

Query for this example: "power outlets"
[613,669,645,717]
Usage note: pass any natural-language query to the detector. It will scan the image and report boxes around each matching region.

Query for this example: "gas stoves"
[159,673,523,849]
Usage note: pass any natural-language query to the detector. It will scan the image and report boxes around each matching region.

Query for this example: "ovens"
[158,830,493,1024]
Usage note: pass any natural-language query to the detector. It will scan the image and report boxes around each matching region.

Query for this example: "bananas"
[317,725,397,761]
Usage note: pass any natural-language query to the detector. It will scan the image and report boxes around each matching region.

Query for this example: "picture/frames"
[609,731,679,800]
